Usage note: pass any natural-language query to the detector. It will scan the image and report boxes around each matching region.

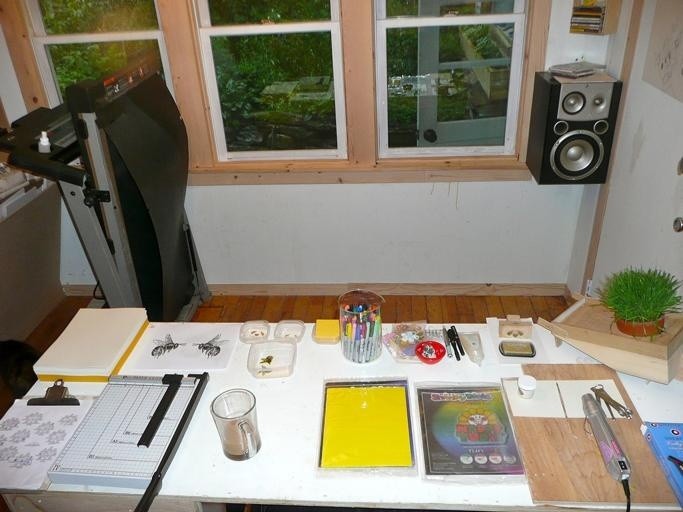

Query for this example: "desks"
[1,307,682,512]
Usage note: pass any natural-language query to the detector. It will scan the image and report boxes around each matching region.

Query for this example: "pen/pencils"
[442,326,465,361]
[340,303,382,363]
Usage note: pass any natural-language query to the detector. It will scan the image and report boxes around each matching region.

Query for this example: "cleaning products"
[38,131,51,154]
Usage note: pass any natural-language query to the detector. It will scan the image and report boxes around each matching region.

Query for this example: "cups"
[208,389,260,462]
[338,288,386,364]
[516,374,537,400]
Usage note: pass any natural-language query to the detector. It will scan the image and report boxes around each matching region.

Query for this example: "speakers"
[526,72,623,185]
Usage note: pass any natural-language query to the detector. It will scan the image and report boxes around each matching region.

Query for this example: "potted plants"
[578,260,683,345]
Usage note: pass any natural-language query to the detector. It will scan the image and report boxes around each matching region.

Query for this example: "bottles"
[37,130,50,152]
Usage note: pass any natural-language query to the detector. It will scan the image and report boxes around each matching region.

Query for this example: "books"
[548,62,597,79]
[640,419,682,509]
